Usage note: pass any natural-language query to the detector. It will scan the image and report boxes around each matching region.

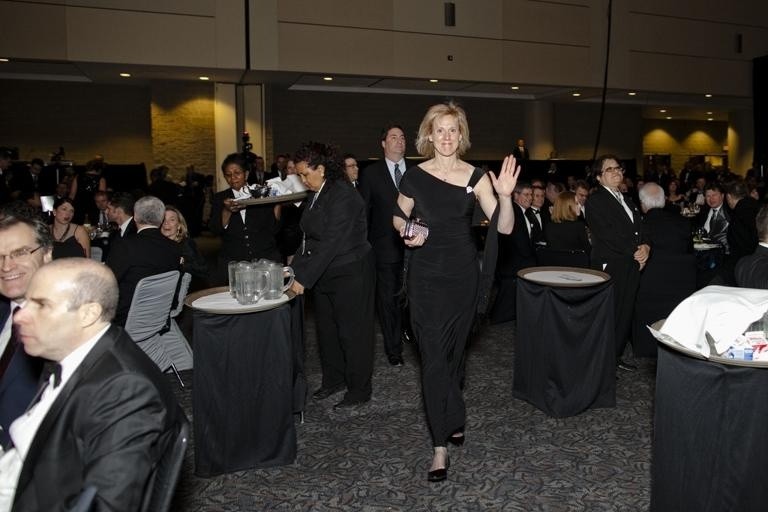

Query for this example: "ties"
[393,164,403,186]
[1,306,22,374]
[710,210,717,233]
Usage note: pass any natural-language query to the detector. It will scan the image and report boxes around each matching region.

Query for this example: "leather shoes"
[428,450,450,482]
[448,428,465,445]
[388,353,403,367]
[333,397,370,410]
[401,326,413,345]
[313,381,345,400]
[616,358,635,373]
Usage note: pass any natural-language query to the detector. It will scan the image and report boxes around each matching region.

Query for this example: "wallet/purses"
[403,219,429,242]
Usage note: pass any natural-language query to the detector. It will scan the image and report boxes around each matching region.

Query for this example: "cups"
[683,208,688,216]
[228,259,295,305]
[88,227,97,241]
[695,208,700,214]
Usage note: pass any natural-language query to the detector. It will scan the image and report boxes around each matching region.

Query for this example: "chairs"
[0,201,193,512]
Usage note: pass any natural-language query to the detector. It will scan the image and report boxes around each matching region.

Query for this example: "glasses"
[606,167,622,172]
[0,245,43,265]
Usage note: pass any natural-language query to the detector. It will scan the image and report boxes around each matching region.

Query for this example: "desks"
[513,265,616,417]
[183,285,300,473]
[647,316,768,512]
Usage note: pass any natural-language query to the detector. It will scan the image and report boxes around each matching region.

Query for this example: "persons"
[498,137,768,372]
[359,125,421,366]
[290,142,375,412]
[392,99,522,482]
[0,153,300,512]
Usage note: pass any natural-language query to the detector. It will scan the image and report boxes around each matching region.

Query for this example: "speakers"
[444,2,456,27]
